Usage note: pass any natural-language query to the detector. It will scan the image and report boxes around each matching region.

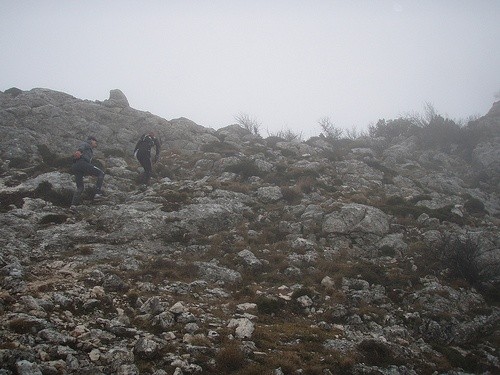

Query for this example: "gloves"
[73,151,82,160]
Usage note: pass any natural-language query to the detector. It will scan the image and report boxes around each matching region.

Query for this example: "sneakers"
[69,208,80,216]
[94,195,110,202]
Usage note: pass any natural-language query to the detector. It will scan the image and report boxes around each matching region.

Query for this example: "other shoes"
[138,184,147,193]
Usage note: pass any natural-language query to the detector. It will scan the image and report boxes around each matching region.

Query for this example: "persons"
[133,132,163,189]
[69,135,108,210]
